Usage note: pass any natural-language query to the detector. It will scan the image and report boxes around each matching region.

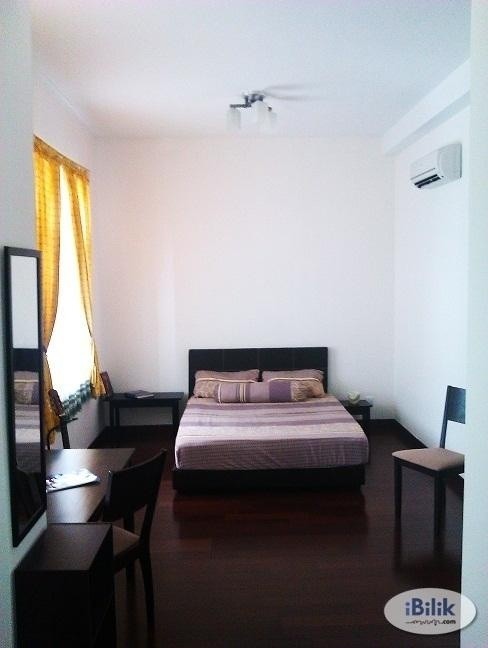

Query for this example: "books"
[46,466,101,494]
[124,390,154,400]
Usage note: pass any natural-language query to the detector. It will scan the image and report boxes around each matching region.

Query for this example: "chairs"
[392,385,466,537]
[102,447,167,625]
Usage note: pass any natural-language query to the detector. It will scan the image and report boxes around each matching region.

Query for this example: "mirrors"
[5,246,48,549]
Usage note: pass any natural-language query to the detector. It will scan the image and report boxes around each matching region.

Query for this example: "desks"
[103,392,183,428]
[47,446,136,588]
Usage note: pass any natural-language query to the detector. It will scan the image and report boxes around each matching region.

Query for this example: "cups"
[347,391,361,405]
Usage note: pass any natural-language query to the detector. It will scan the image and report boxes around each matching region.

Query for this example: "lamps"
[225,95,279,131]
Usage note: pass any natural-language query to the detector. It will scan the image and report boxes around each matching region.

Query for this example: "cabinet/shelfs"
[13,522,115,648]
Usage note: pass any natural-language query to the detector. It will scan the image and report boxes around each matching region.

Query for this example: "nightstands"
[340,399,372,435]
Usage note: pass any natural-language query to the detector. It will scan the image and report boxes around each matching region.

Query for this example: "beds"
[172,347,369,495]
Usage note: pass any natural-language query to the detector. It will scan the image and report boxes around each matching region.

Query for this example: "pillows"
[262,369,325,398]
[194,370,260,399]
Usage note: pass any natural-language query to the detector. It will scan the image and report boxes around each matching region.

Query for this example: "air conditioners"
[409,142,462,190]
